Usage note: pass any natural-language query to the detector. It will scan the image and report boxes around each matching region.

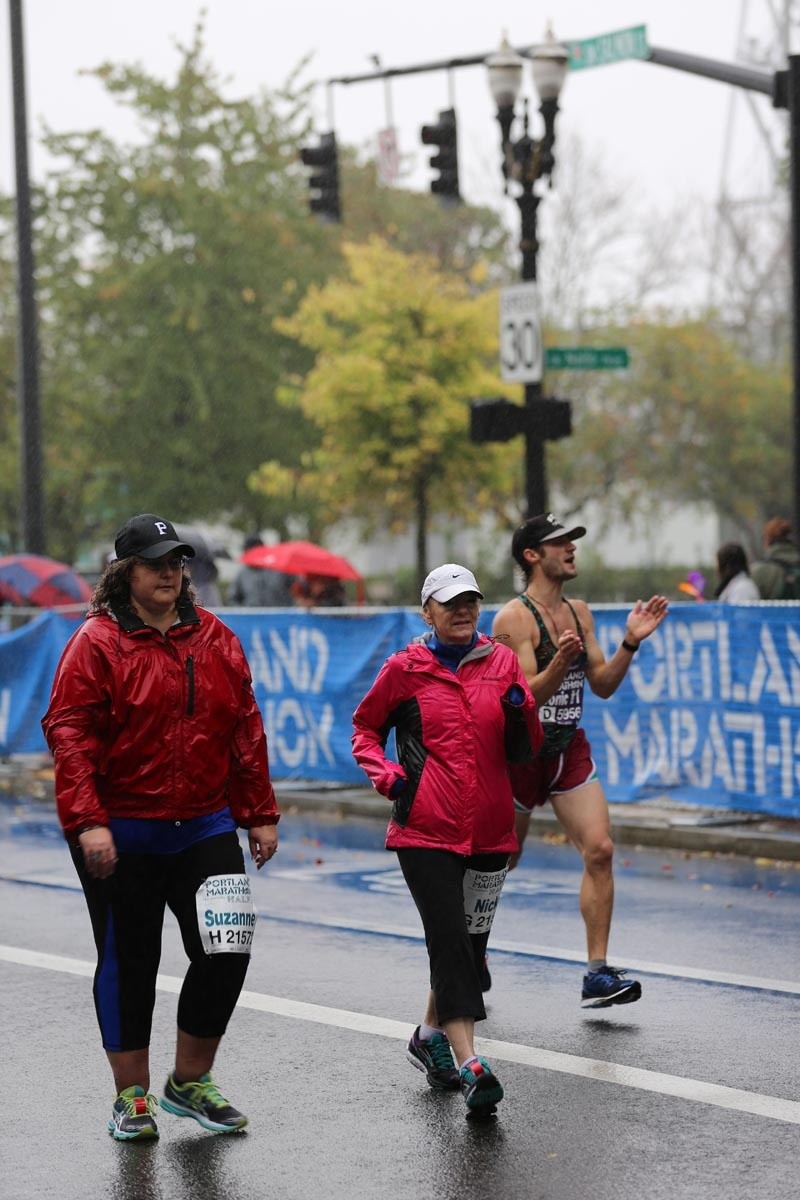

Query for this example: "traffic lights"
[418,109,458,200]
[300,132,342,230]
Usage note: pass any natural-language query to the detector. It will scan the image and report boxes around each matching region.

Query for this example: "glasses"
[133,557,186,570]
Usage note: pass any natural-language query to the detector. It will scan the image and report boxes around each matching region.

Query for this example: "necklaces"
[525,588,559,641]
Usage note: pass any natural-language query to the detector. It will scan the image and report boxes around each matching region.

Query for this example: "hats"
[511,512,587,561]
[421,564,485,608]
[114,513,196,559]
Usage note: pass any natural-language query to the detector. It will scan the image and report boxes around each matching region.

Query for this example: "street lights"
[486,19,572,586]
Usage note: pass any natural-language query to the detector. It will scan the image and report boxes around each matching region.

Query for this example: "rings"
[90,855,99,862]
[275,851,277,852]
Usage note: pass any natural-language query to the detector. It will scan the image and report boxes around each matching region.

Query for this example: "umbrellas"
[237,541,362,602]
[0,556,93,606]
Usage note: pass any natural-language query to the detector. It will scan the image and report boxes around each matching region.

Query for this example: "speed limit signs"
[495,282,542,385]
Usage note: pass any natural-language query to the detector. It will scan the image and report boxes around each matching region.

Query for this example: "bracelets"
[621,639,638,651]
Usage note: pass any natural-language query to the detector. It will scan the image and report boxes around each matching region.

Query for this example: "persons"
[478,514,668,1010]
[41,522,281,1141]
[350,564,544,1114]
[0,517,798,611]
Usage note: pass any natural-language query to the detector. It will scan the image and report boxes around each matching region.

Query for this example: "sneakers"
[407,1025,459,1088]
[580,963,641,1009]
[159,1069,249,1131]
[459,1054,504,1114]
[107,1085,160,1140]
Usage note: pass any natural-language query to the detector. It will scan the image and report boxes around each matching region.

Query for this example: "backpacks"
[773,558,800,599]
